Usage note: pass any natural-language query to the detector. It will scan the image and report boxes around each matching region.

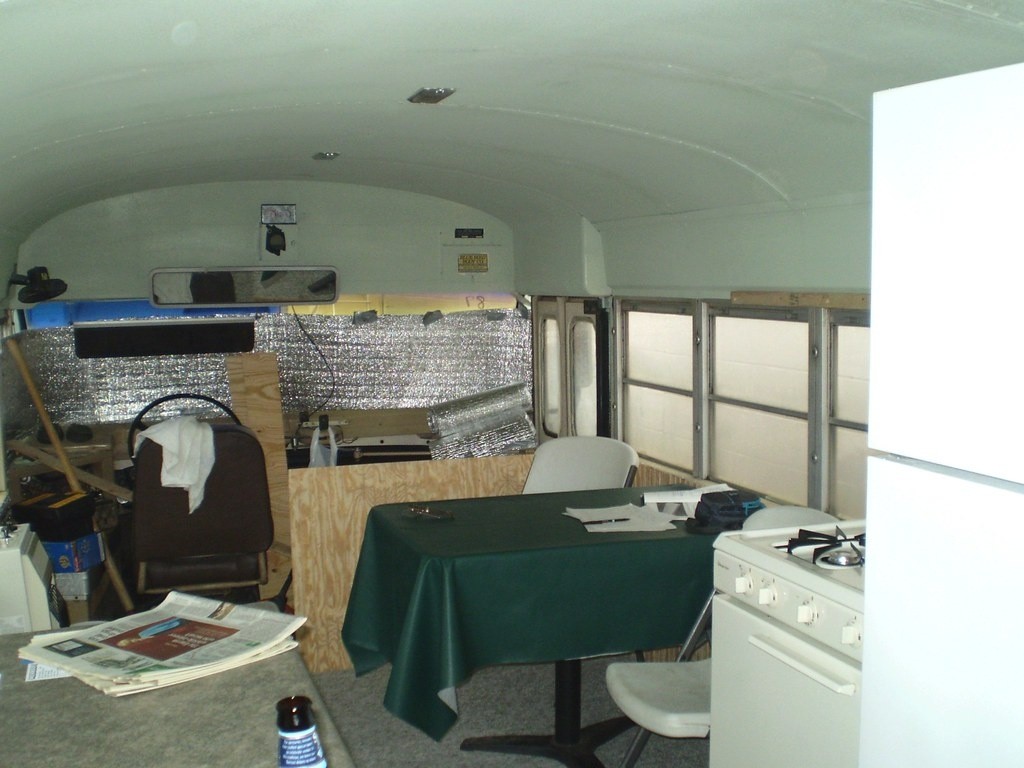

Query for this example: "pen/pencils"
[582,517,629,524]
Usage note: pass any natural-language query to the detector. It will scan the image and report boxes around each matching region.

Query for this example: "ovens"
[707,551,860,768]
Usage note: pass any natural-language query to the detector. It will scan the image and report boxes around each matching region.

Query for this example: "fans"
[11,266,68,303]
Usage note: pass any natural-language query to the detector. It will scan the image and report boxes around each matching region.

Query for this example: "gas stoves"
[711,516,866,614]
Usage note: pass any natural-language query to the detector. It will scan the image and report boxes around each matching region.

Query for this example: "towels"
[129,412,214,515]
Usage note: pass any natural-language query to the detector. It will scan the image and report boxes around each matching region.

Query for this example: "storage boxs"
[39,531,105,604]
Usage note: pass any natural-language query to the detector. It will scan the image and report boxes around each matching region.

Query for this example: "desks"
[342,483,766,768]
[0,599,359,768]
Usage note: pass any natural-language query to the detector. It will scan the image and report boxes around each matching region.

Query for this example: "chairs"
[525,435,639,663]
[130,419,275,617]
[604,589,712,766]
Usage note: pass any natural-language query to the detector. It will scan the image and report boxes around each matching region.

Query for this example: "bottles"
[309,414,337,467]
[275,696,328,768]
